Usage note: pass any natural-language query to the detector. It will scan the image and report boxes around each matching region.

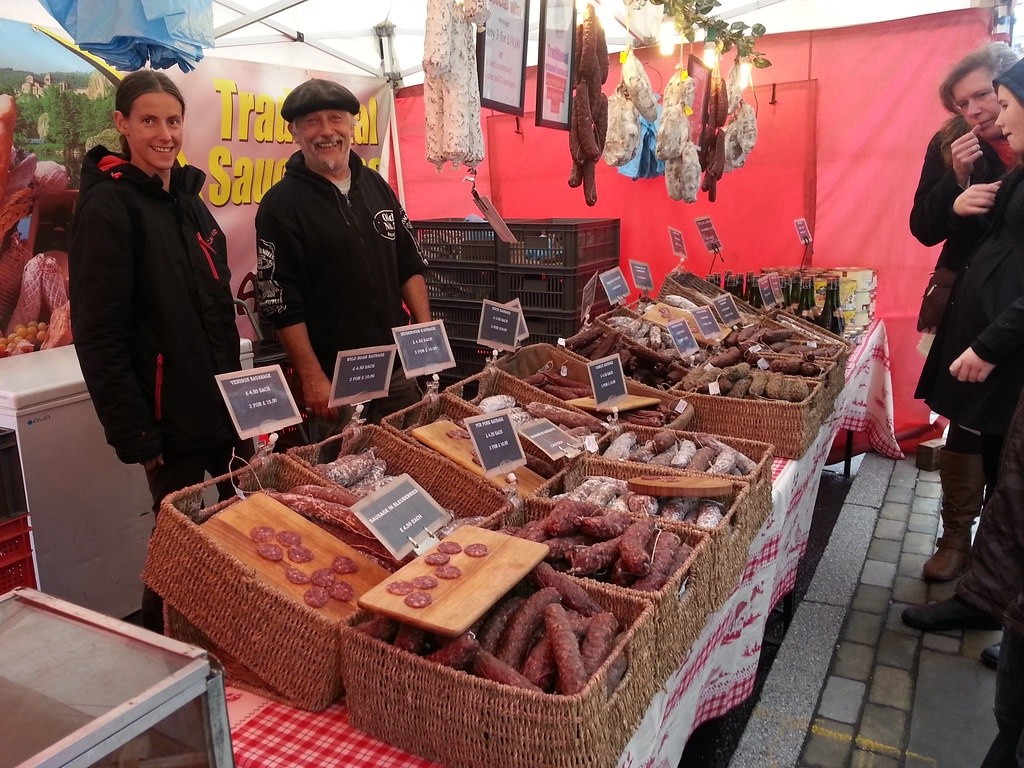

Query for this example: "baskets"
[139,215,857,768]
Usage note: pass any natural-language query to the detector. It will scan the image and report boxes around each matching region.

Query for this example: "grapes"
[0,321,48,352]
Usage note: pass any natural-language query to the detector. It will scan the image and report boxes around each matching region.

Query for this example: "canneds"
[814,266,877,355]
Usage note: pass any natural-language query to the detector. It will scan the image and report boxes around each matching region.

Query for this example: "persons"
[67,70,244,637]
[901,43,1024,768]
[255,78,432,464]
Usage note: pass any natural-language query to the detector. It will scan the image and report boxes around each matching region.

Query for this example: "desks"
[223,316,906,768]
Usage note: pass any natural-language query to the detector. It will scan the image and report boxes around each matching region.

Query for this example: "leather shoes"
[901,595,1003,632]
[981,642,1002,668]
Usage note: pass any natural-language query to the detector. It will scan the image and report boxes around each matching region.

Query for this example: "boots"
[923,446,986,580]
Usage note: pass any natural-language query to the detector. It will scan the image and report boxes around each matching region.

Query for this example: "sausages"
[247,360,758,696]
[421,0,491,166]
[566,4,609,206]
[563,294,842,404]
[697,75,729,200]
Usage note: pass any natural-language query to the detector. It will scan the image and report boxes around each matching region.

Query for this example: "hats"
[281,78,360,122]
[992,58,1024,101]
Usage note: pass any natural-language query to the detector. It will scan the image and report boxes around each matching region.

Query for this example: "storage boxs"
[0,587,237,768]
[413,216,620,398]
